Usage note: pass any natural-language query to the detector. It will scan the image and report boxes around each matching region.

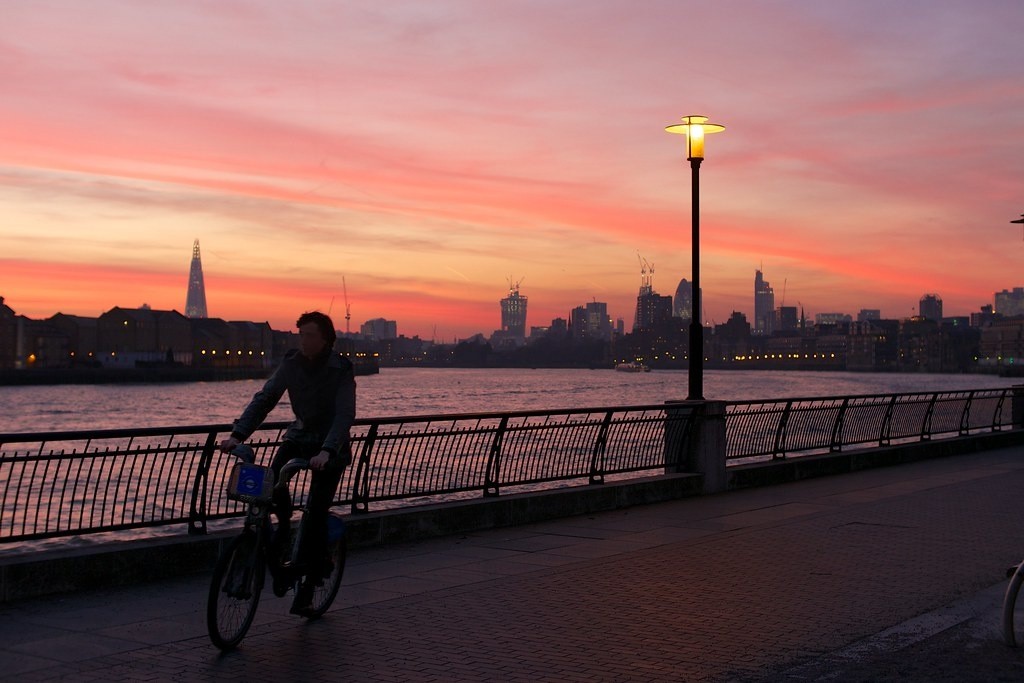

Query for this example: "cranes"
[342,277,352,332]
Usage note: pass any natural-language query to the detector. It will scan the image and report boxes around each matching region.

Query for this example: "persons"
[220,311,356,615]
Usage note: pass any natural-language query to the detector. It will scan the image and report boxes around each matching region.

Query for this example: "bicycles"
[207,446,350,652]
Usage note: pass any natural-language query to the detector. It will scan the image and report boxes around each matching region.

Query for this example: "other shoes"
[273,519,291,552]
[291,575,316,611]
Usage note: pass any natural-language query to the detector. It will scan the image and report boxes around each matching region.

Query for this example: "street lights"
[667,115,725,399]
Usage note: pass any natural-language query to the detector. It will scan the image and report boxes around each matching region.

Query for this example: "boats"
[615,359,651,372]
